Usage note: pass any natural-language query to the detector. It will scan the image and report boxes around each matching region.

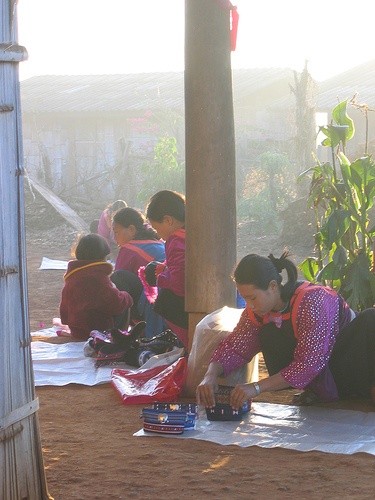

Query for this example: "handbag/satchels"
[152,288,188,328]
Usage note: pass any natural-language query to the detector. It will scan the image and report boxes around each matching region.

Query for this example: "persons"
[91,199,168,321]
[144,189,246,351]
[59,233,133,341]
[195,249,375,411]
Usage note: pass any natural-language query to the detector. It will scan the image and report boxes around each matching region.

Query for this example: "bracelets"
[254,382,260,395]
